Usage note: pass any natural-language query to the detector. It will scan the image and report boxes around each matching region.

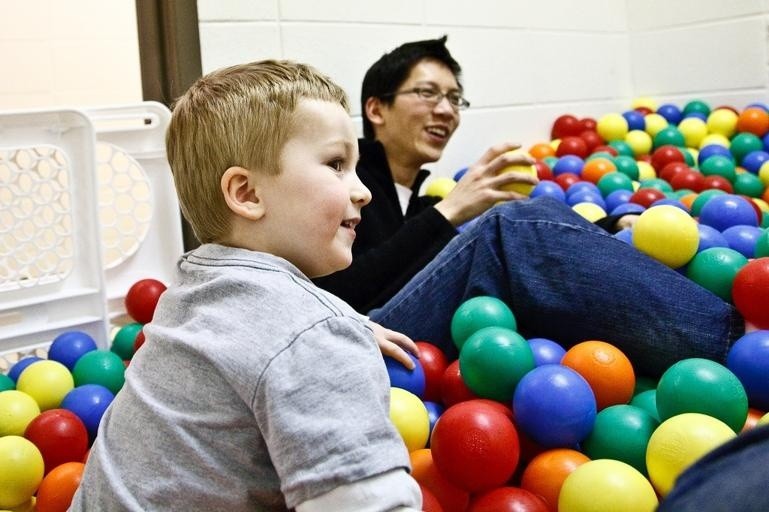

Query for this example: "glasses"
[382,84,471,111]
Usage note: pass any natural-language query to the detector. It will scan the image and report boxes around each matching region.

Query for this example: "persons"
[320,34,745,366]
[67,59,424,510]
